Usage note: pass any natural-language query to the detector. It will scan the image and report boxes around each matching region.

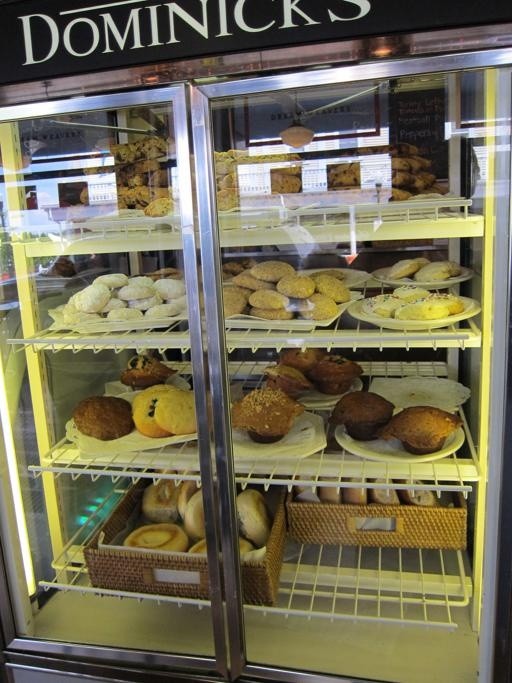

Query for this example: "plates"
[65,390,198,450]
[232,411,329,463]
[50,303,175,334]
[346,293,482,331]
[371,265,474,291]
[295,268,371,289]
[295,376,364,408]
[335,407,465,463]
[225,291,362,333]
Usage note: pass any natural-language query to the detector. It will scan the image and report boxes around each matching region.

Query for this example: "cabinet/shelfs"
[0,22,512,683]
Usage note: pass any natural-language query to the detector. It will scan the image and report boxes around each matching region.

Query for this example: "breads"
[312,356,365,395]
[215,260,352,320]
[385,406,463,454]
[330,391,396,440]
[121,354,178,387]
[383,255,462,282]
[132,384,176,438]
[125,471,272,558]
[231,385,306,444]
[369,282,465,320]
[78,136,176,217]
[61,272,186,323]
[215,147,305,210]
[279,347,323,373]
[265,365,311,397]
[325,140,442,203]
[152,390,197,435]
[297,475,440,507]
[74,396,131,440]
